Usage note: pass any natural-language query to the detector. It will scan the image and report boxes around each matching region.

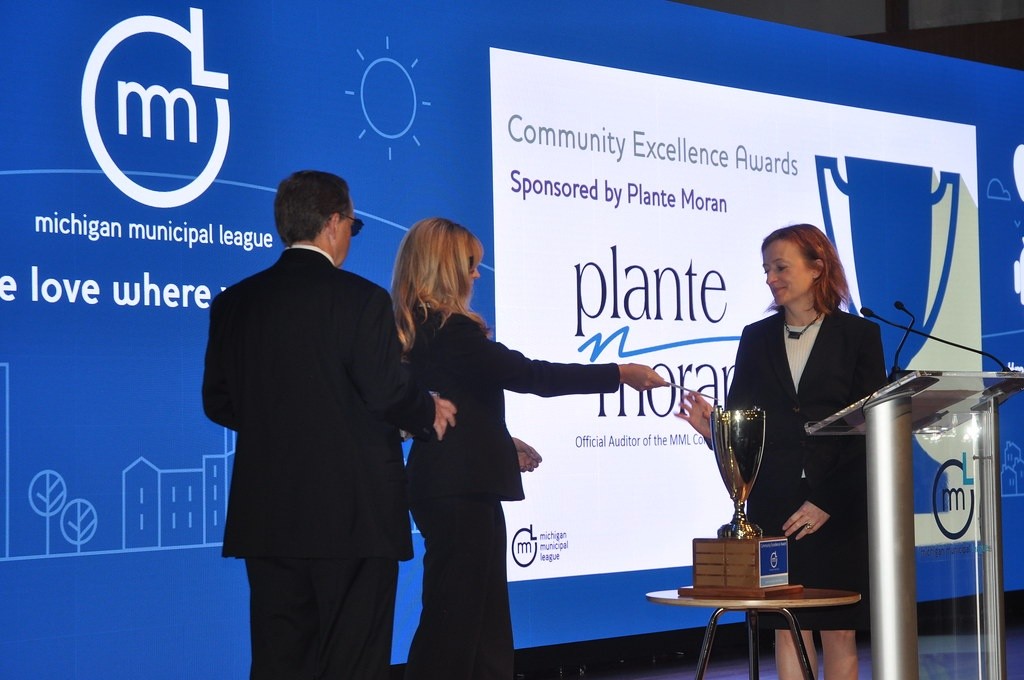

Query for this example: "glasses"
[327,211,364,237]
[466,255,476,270]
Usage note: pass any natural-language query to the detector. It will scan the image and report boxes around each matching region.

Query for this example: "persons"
[674,224,888,680]
[201,170,458,679]
[390,218,669,680]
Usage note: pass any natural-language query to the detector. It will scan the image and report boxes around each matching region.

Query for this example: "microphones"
[860,301,1019,386]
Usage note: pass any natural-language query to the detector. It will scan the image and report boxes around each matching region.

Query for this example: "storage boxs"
[693,536,790,588]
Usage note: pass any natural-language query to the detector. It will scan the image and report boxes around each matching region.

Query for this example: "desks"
[647,587,863,680]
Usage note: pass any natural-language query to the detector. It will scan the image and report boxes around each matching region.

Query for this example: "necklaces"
[783,312,823,339]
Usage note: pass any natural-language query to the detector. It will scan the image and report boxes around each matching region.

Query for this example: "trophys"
[678,405,803,597]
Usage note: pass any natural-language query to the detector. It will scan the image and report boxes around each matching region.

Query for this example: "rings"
[806,523,812,530]
